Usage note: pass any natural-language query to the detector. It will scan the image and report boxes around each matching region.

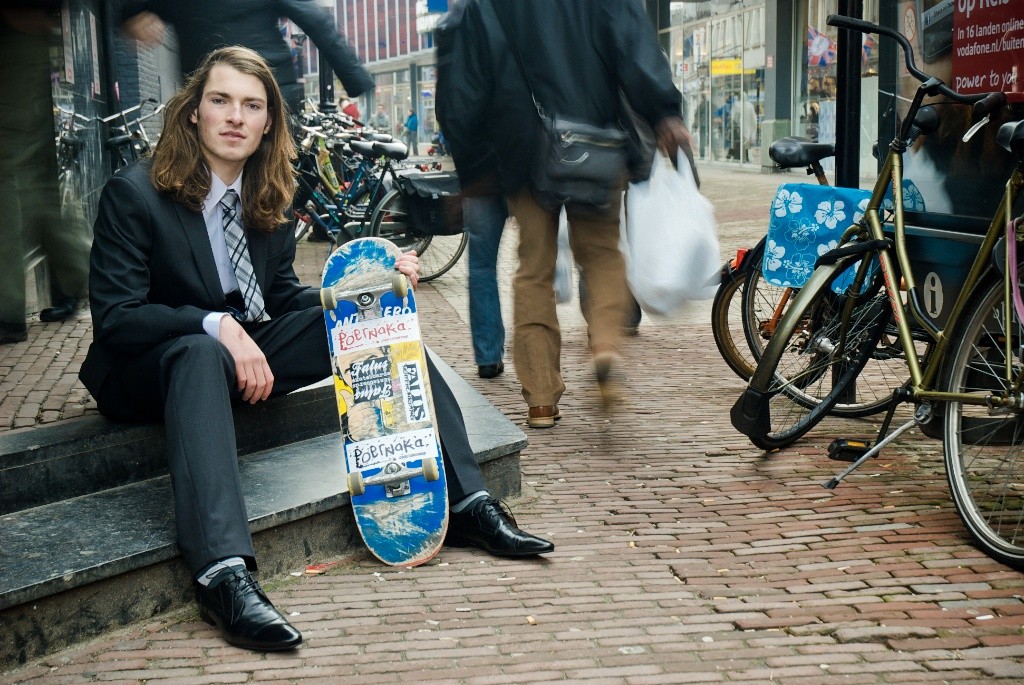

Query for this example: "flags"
[807,26,873,69]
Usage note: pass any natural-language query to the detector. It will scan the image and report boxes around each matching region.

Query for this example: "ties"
[219,191,271,326]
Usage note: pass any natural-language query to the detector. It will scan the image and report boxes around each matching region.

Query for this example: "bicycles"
[96,81,165,172]
[710,13,1022,563]
[49,101,94,246]
[279,88,469,289]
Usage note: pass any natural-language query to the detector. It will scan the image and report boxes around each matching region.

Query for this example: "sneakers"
[478,362,506,378]
[0,324,30,345]
[40,301,79,322]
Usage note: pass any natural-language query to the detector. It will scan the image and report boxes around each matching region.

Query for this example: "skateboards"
[320,237,449,568]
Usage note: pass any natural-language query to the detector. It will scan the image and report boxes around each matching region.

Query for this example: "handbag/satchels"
[615,87,659,187]
[533,107,634,213]
[621,144,723,315]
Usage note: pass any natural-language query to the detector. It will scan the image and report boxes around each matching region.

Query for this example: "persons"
[400,107,420,158]
[433,0,512,382]
[463,1,695,427]
[74,43,560,651]
[689,74,835,162]
[142,0,382,273]
[338,95,363,132]
[0,0,173,348]
[367,105,390,136]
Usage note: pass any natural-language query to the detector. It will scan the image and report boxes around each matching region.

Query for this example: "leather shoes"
[527,403,560,428]
[444,494,556,556]
[195,564,303,649]
